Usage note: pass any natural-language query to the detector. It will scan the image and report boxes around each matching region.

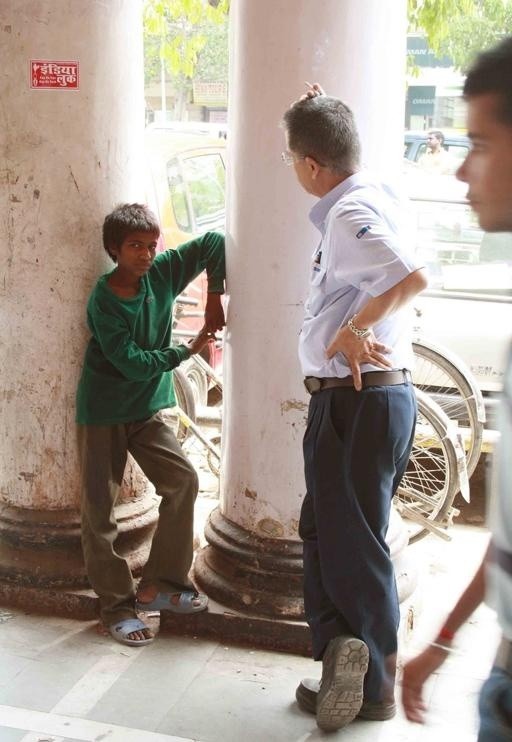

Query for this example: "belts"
[303,368,412,395]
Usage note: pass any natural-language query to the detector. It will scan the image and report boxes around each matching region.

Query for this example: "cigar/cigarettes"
[305,81,319,95]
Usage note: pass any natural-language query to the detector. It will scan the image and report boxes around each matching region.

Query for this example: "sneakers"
[316,633,371,733]
[295,678,395,722]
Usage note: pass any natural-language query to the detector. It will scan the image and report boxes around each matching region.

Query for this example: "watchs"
[346,315,372,339]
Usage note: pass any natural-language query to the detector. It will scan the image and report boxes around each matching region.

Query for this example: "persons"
[75,204,225,644]
[427,130,448,156]
[281,81,428,733]
[401,34,512,741]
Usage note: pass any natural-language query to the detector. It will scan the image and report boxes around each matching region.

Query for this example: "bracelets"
[436,625,455,640]
[431,641,457,653]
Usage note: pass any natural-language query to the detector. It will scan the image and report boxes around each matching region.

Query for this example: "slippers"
[106,616,154,648]
[134,590,209,614]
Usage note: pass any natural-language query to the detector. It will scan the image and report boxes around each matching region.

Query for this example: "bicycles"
[158,287,488,521]
[158,294,473,550]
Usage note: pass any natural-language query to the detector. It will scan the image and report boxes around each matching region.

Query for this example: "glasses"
[279,149,329,171]
